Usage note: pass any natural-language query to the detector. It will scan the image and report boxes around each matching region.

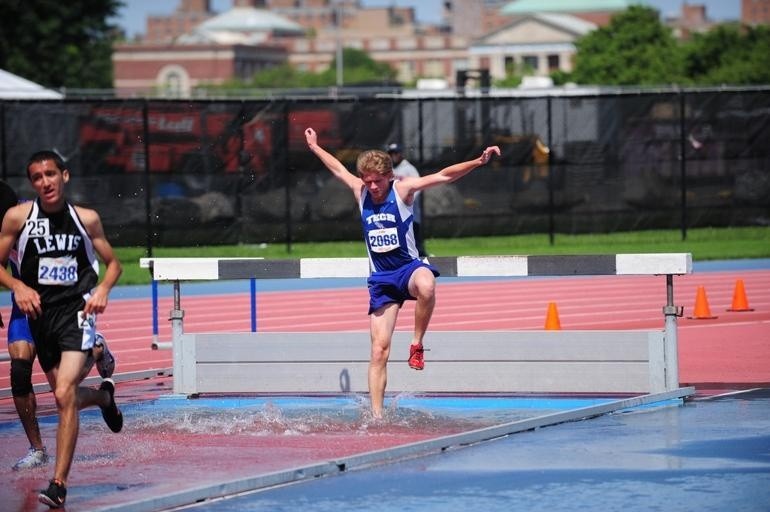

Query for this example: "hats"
[387,143,399,153]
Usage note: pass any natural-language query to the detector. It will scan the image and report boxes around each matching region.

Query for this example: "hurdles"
[140,252,693,400]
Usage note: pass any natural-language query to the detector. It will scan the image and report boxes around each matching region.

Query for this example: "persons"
[0,178,114,471]
[0,150,124,507]
[303,128,501,422]
[385,143,426,256]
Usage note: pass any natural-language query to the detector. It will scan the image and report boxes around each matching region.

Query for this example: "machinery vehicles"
[618,102,770,214]
[456,67,566,188]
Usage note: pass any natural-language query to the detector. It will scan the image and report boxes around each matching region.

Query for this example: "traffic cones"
[546,304,565,329]
[727,279,754,312]
[689,285,720,319]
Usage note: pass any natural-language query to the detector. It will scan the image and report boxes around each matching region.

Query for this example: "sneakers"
[38,479,66,507]
[94,333,122,432]
[13,447,48,471]
[409,343,424,370]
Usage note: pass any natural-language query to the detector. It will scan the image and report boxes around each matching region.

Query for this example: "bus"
[71,98,406,199]
